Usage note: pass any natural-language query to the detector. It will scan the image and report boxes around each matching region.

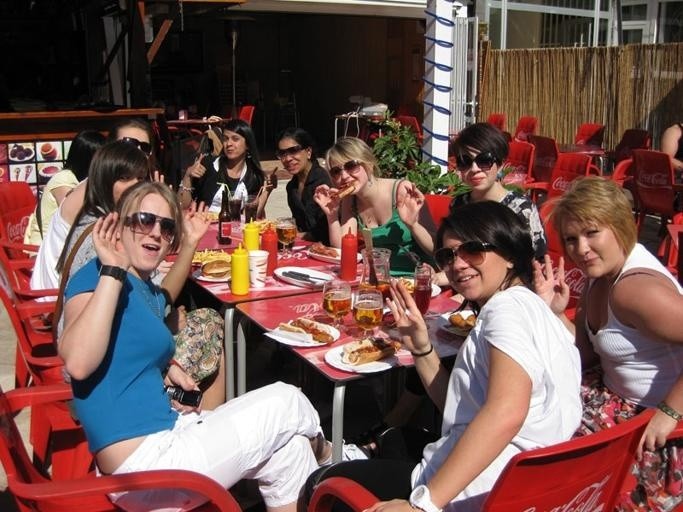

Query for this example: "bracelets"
[178,180,195,193]
[98,263,127,283]
[409,343,434,358]
[656,399,681,423]
[169,358,182,369]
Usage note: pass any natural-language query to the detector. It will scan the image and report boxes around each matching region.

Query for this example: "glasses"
[433,241,496,271]
[274,145,306,160]
[118,137,152,156]
[329,160,360,179]
[123,212,176,241]
[455,151,499,171]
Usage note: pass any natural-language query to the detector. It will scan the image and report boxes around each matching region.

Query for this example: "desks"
[165,116,237,155]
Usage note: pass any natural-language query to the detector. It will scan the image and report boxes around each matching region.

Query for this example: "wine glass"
[321,280,351,333]
[276,217,296,258]
[352,291,383,339]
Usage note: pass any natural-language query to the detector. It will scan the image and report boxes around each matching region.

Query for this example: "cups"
[248,249,268,288]
[413,266,433,315]
[242,195,258,223]
[228,190,243,222]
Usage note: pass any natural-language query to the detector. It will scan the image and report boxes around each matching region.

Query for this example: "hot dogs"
[278,318,334,344]
[449,312,476,332]
[341,338,409,366]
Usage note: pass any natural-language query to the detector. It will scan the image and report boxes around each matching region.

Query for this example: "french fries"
[192,248,231,264]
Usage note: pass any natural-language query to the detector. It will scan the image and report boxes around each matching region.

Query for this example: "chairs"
[362,111,681,335]
[298,403,658,511]
[1,181,241,508]
[235,105,254,125]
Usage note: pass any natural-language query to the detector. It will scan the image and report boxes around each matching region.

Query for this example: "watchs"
[404,483,442,512]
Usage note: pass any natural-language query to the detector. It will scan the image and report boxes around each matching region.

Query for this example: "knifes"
[281,269,328,284]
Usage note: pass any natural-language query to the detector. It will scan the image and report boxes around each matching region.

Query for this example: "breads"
[398,276,415,292]
[333,185,355,199]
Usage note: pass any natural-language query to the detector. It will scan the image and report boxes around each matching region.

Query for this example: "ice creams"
[14,168,20,182]
[24,166,32,181]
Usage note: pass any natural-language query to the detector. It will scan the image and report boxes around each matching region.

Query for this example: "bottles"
[230,242,249,296]
[351,248,395,328]
[243,218,258,252]
[216,191,232,245]
[259,223,278,276]
[339,227,357,280]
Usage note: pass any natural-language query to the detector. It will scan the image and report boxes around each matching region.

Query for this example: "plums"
[11,146,33,160]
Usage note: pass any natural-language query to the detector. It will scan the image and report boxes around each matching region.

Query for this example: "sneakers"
[318,441,368,466]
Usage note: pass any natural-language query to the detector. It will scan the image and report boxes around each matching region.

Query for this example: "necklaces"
[129,268,162,319]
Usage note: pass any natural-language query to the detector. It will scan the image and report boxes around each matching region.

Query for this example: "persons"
[531,172,682,511]
[270,125,339,247]
[369,120,549,433]
[20,130,108,278]
[308,135,440,423]
[293,193,584,512]
[175,119,278,224]
[27,116,172,331]
[658,120,682,212]
[58,180,380,511]
[49,136,228,426]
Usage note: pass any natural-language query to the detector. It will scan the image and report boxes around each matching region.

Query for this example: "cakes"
[38,166,61,183]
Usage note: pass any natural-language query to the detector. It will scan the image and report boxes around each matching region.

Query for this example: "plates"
[436,311,478,337]
[325,344,400,373]
[8,148,35,161]
[38,165,60,177]
[193,210,219,224]
[273,323,340,347]
[394,279,441,299]
[272,266,332,288]
[191,267,231,282]
[306,247,362,263]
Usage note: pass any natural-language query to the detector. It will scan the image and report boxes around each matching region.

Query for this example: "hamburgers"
[200,262,233,279]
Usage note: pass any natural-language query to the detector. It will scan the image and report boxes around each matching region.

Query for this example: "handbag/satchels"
[167,307,224,382]
